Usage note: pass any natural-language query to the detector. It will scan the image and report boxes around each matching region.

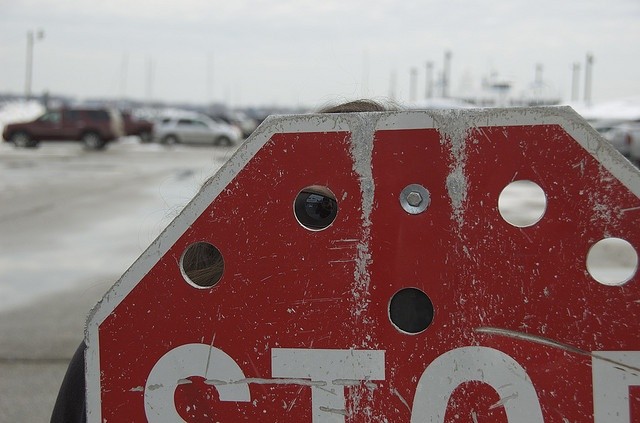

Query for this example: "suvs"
[4,106,121,150]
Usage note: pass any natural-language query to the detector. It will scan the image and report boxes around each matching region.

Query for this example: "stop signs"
[82,104,640,423]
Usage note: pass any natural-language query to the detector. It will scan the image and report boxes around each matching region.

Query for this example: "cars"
[121,105,155,143]
[152,110,242,147]
